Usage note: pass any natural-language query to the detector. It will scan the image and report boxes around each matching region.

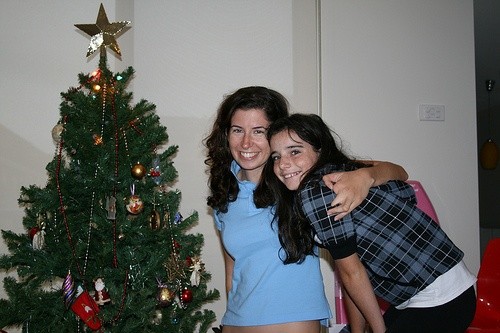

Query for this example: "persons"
[264,112,479,333]
[201,86,408,333]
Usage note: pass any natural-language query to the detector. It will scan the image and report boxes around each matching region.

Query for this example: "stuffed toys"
[93,278,110,305]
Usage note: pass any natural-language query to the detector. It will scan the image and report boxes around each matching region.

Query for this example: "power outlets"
[420,105,445,121]
